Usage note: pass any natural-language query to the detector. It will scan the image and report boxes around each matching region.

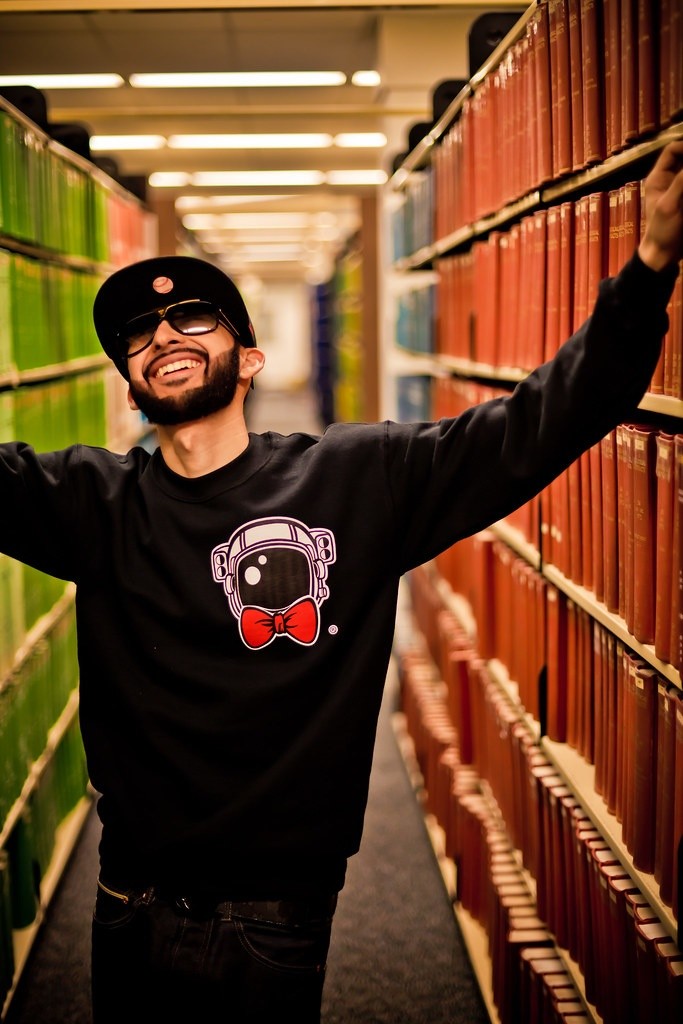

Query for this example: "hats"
[92,256,258,381]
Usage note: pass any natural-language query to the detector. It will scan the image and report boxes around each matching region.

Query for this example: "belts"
[96,866,338,929]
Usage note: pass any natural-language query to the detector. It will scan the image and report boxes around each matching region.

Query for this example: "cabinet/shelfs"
[0,2,683,1024]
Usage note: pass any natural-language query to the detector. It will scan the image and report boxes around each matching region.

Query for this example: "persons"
[0,140,682,1024]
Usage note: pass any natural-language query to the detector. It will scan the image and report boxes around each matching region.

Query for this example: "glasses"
[115,298,255,389]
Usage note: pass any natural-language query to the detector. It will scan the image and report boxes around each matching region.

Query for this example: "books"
[0,112,151,1008]
[311,1,683,1024]
